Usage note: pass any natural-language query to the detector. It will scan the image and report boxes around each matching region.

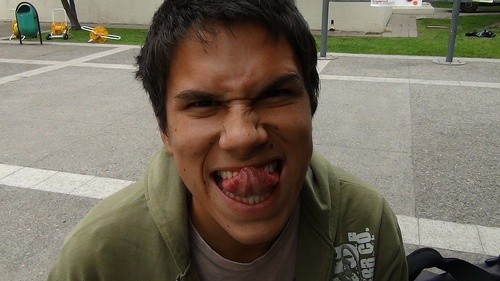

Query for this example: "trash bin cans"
[15,2,42,45]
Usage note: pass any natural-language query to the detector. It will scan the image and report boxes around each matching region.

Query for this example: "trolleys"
[8,9,26,41]
[81,26,121,43]
[46,8,70,40]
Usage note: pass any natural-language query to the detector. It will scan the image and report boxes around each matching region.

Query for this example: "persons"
[42,1,409,281]
